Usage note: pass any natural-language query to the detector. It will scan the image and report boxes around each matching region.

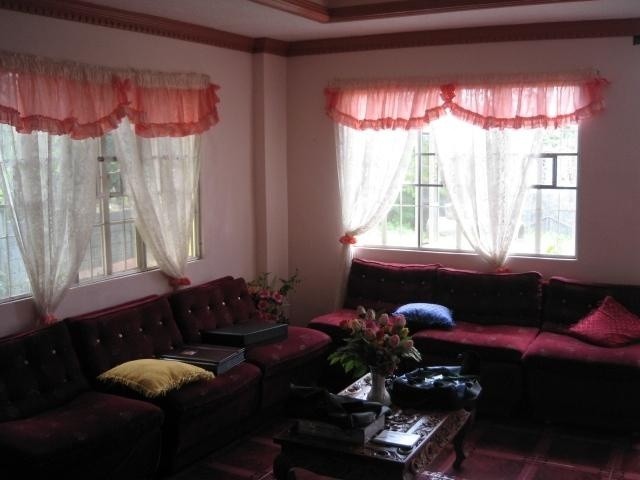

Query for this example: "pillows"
[561,295,640,352]
[390,299,462,331]
[92,358,220,400]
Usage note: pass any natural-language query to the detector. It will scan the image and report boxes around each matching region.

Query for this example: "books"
[161,345,245,376]
[374,429,420,448]
[200,322,289,348]
[299,414,385,444]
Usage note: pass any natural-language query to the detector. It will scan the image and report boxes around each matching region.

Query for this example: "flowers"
[326,304,425,381]
[244,268,303,326]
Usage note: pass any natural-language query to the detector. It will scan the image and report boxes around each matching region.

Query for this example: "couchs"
[308,255,640,439]
[0,275,334,477]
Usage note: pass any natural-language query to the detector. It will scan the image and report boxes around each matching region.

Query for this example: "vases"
[364,363,394,408]
